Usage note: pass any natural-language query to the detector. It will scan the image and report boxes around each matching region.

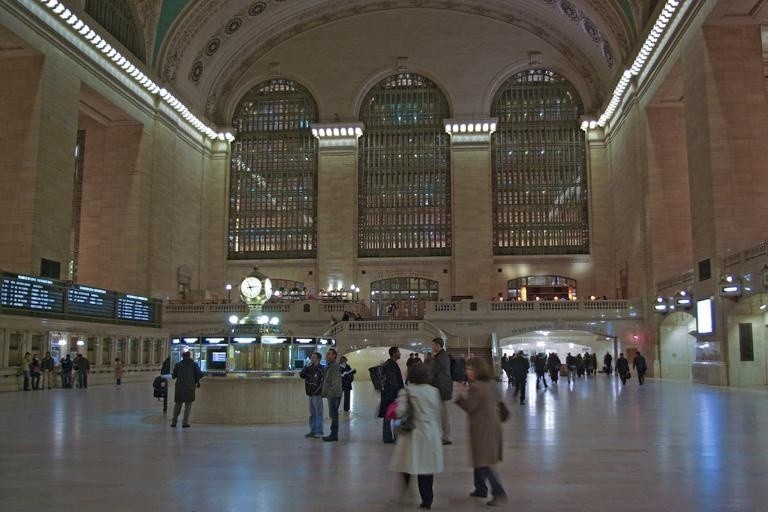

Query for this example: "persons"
[340,356,353,411]
[21,352,90,391]
[633,351,647,385]
[567,352,596,383]
[322,348,342,441]
[170,352,203,427]
[604,353,614,376]
[161,357,170,413]
[116,357,122,385]
[494,289,608,310]
[503,350,561,406]
[272,287,355,306]
[376,336,475,445]
[300,352,326,438]
[389,364,450,511]
[452,356,510,506]
[615,353,631,385]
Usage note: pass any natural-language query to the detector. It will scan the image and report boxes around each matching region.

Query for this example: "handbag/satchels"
[399,389,414,432]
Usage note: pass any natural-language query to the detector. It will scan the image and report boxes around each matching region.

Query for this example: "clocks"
[264,278,273,299]
[240,276,262,299]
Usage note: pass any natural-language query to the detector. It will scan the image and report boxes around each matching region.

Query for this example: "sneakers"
[305,432,313,438]
[313,432,322,438]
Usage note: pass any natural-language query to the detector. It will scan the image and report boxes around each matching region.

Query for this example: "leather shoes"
[322,435,338,442]
[419,502,432,510]
[469,492,488,498]
[486,500,506,506]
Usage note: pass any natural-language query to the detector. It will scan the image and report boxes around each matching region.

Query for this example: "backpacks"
[448,355,468,383]
[368,359,392,391]
